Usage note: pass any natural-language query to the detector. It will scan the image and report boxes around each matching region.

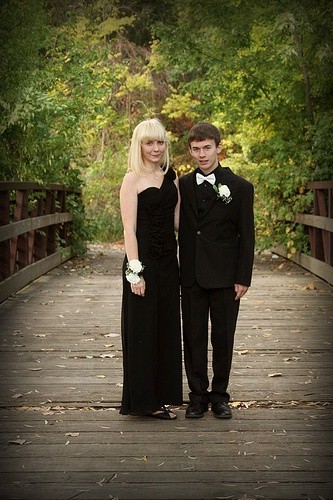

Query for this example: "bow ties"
[196,173,216,185]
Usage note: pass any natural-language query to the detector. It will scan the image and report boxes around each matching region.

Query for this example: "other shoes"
[143,405,176,419]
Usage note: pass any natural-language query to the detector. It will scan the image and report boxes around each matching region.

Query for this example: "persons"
[178,121,256,419]
[119,119,183,420]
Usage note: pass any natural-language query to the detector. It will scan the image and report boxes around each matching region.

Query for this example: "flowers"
[212,182,232,204]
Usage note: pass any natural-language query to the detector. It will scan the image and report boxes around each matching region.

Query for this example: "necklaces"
[152,175,162,179]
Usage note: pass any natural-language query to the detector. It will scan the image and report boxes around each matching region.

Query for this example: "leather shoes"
[211,400,232,418]
[185,400,208,418]
